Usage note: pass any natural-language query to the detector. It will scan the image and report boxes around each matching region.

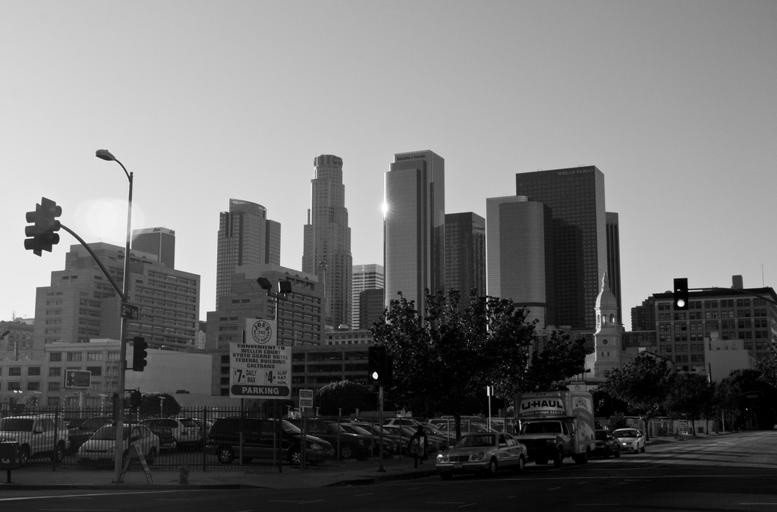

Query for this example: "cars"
[595,428,646,458]
[435,433,528,478]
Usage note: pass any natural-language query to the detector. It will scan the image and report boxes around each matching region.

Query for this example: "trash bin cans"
[1,441,20,473]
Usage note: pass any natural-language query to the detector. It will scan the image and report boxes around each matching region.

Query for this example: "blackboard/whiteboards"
[135,444,152,475]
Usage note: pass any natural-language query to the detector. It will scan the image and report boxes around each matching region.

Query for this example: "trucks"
[514,391,595,465]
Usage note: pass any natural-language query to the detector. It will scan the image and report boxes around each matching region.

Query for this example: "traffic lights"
[675,278,688,310]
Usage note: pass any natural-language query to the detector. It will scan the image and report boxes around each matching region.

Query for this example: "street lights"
[257,278,291,465]
[95,149,133,486]
